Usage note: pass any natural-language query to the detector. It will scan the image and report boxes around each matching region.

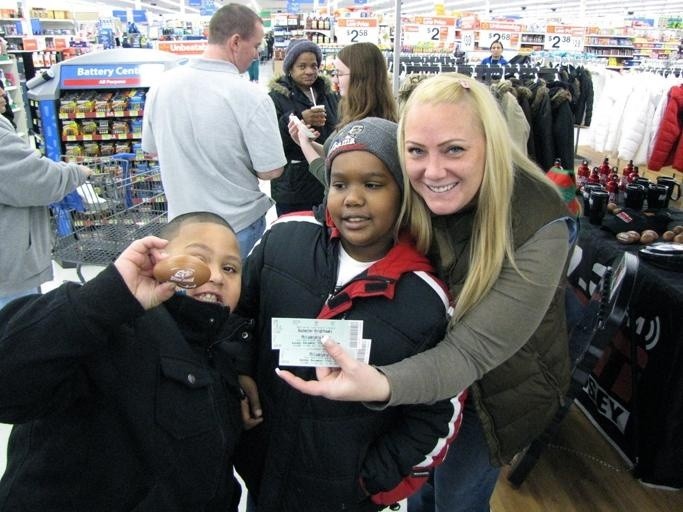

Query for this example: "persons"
[480,41,508,66]
[265,33,274,59]
[288,43,400,228]
[247,55,259,83]
[267,38,340,220]
[275,71,578,512]
[0,212,249,512]
[0,89,94,310]
[233,116,463,512]
[141,4,287,269]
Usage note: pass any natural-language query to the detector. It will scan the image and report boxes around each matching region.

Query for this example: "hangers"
[469,62,573,85]
[628,60,683,80]
[398,55,460,74]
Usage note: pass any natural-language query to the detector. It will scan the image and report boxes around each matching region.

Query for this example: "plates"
[638,241,683,273]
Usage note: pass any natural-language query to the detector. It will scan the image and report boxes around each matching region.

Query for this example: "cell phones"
[288,113,319,141]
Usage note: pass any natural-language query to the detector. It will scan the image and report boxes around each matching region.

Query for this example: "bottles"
[306,16,330,30]
[307,33,331,43]
[588,37,632,46]
[554,158,639,204]
[32,50,57,67]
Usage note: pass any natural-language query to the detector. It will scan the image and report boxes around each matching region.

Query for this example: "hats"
[326,117,404,194]
[282,39,322,74]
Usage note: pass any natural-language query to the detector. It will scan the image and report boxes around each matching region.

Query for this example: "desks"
[563,203,682,493]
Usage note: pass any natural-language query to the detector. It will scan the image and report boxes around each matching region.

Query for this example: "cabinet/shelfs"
[273,25,304,79]
[55,88,167,234]
[0,7,77,52]
[7,49,90,91]
[0,53,29,145]
[305,14,336,44]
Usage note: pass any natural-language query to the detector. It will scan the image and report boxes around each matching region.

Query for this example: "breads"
[607,201,622,213]
[615,224,682,244]
[152,254,212,291]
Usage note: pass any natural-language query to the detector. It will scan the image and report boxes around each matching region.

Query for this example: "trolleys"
[45,154,167,281]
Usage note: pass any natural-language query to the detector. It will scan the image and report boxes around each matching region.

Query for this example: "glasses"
[335,72,352,80]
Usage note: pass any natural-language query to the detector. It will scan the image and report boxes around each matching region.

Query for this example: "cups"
[588,48,633,56]
[582,176,682,226]
[311,104,325,127]
